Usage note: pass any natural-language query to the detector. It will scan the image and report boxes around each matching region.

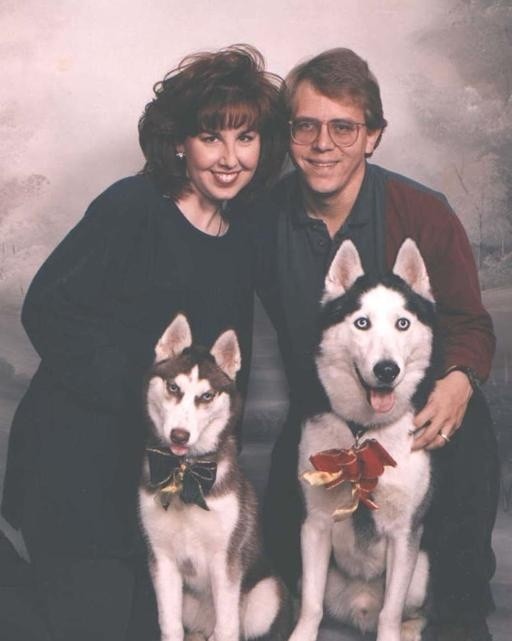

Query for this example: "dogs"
[286,236,442,641]
[134,311,289,641]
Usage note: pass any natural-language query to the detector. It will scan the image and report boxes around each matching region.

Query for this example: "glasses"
[288,116,366,148]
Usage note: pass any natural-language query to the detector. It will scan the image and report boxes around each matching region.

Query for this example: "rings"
[438,431,451,444]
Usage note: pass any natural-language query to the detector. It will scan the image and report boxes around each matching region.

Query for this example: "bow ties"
[146,448,218,509]
[302,437,397,523]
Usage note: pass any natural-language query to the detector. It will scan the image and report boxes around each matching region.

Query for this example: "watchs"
[442,365,480,393]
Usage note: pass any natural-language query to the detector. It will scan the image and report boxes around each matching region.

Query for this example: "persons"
[0,45,298,640]
[253,47,499,640]
